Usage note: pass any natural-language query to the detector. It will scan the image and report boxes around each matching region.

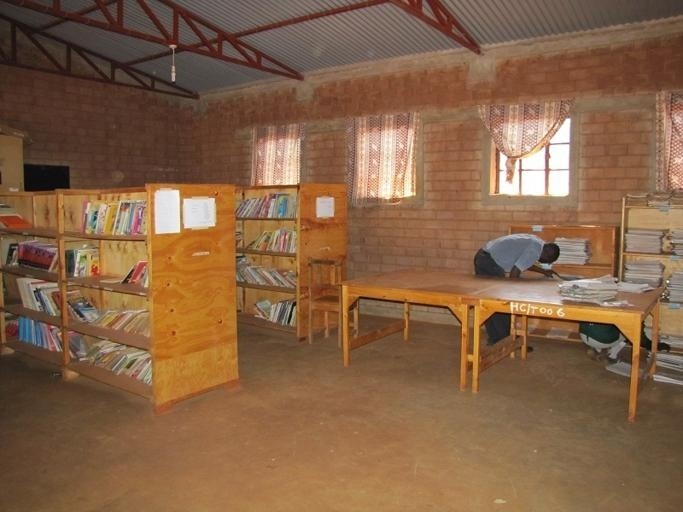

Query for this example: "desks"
[459,277,667,423]
[337,265,513,393]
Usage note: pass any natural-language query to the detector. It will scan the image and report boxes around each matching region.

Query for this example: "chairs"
[305,255,361,348]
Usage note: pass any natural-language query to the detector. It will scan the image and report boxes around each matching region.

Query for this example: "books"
[5,240,59,274]
[66,289,152,339]
[253,299,297,330]
[622,225,682,254]
[235,228,297,253]
[236,254,297,289]
[603,360,643,378]
[551,236,592,269]
[625,188,682,209]
[64,248,148,288]
[66,330,153,387]
[15,278,62,317]
[622,262,682,303]
[81,201,147,235]
[648,330,682,385]
[16,317,63,354]
[559,274,652,309]
[236,192,296,218]
[0,202,32,229]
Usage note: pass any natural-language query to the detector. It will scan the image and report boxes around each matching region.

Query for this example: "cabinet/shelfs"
[618,194,683,354]
[234,183,349,345]
[508,222,619,343]
[0,183,240,415]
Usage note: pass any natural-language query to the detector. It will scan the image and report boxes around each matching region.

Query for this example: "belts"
[478,249,488,254]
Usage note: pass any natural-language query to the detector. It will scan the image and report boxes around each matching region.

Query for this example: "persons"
[472,230,559,343]
[577,317,670,362]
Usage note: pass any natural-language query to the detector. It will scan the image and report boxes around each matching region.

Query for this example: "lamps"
[169,44,176,82]
